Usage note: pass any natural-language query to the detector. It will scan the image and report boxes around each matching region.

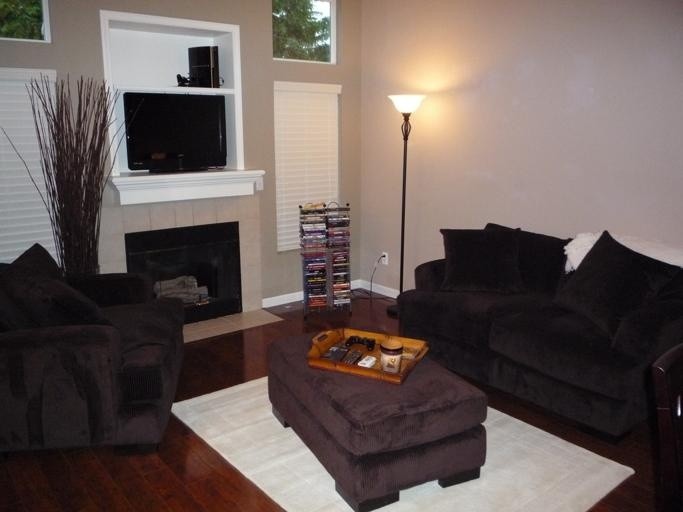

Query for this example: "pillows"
[552,229,681,332]
[441,225,524,293]
[2,262,111,326]
[486,222,572,293]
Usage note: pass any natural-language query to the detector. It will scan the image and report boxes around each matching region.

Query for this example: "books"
[298,207,352,317]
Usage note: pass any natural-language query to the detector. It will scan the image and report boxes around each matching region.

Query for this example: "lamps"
[385,93,426,321]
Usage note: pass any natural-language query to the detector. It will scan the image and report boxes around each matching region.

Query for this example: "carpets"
[172,374,636,511]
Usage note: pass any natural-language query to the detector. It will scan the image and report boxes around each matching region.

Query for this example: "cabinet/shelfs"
[96,7,265,207]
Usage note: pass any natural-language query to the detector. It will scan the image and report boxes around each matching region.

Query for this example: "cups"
[379,340,404,375]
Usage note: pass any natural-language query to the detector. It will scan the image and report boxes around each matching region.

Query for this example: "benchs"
[267,328,489,512]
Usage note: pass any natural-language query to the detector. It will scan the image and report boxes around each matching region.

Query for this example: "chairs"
[0,243,184,452]
[398,259,682,434]
[650,345,683,507]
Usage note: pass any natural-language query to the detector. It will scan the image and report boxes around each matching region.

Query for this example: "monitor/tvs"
[122,92,229,174]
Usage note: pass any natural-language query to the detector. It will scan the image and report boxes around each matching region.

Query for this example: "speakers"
[188,45,220,88]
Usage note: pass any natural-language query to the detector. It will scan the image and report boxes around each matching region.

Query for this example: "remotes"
[344,349,363,366]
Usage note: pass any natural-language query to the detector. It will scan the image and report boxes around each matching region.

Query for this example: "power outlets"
[381,251,389,265]
